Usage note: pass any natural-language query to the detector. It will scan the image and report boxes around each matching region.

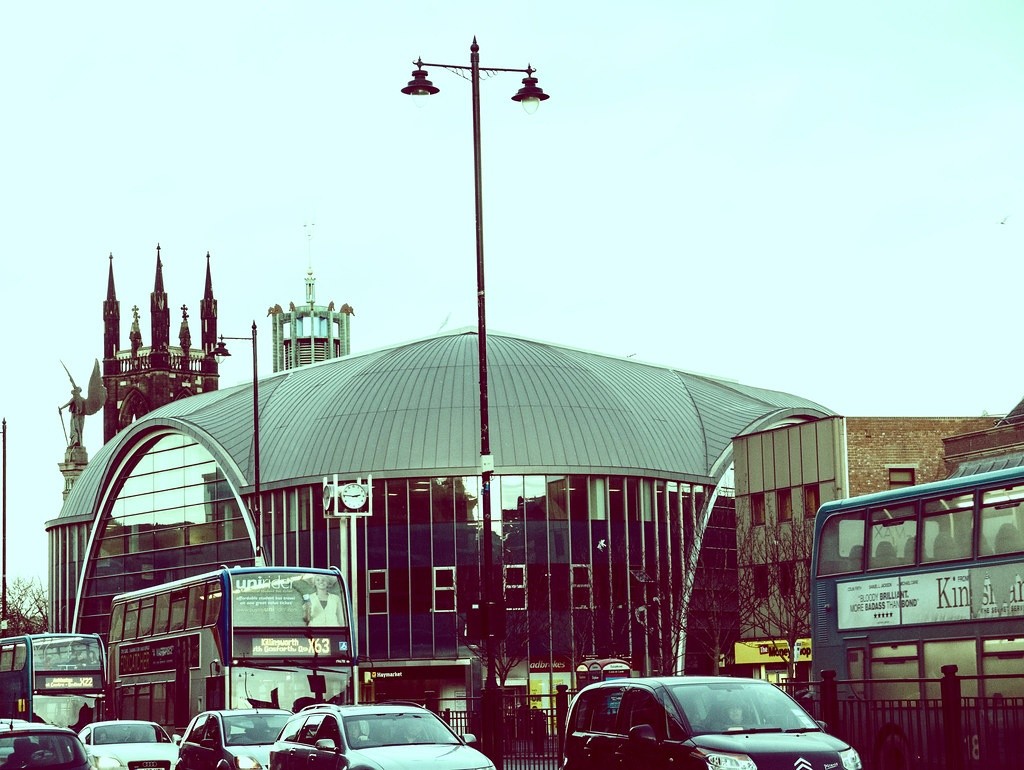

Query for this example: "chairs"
[844,523,1022,570]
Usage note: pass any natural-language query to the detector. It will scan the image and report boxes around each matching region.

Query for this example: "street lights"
[399,39,550,758]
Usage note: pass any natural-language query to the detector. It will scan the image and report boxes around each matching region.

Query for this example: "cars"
[0,713,93,770]
[77,717,181,770]
[267,700,497,769]
[559,669,861,770]
[172,707,292,770]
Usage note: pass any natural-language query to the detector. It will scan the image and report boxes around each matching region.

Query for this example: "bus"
[0,629,110,730]
[107,565,367,733]
[802,466,1024,770]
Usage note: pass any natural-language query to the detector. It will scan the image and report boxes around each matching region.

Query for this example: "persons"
[224,719,234,744]
[532,713,547,757]
[94,727,151,743]
[300,574,345,627]
[67,650,100,670]
[722,695,750,728]
[396,717,428,744]
[345,717,371,745]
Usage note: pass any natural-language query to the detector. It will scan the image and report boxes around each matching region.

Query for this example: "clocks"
[323,485,334,511]
[340,483,367,509]
